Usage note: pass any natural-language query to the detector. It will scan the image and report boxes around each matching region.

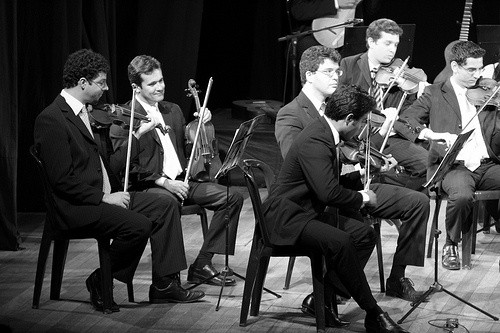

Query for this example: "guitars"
[459,0,473,41]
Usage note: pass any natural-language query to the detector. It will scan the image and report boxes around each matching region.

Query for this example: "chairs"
[29,145,328,333]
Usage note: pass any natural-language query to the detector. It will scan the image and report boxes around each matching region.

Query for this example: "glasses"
[315,69,344,76]
[459,62,486,74]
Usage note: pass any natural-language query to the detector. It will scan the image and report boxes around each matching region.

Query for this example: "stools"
[461,189,500,269]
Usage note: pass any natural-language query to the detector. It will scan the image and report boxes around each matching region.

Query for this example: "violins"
[87,99,171,136]
[377,56,433,95]
[367,107,422,134]
[339,137,405,174]
[185,78,222,182]
[465,79,500,107]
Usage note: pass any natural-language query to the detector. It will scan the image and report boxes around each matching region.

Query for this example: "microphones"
[348,18,363,24]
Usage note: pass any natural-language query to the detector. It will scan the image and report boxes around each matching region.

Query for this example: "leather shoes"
[306,298,350,327]
[386,278,424,300]
[442,244,460,270]
[149,280,204,304]
[365,312,408,333]
[86,269,119,311]
[187,263,236,285]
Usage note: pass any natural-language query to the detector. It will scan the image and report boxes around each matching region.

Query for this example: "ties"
[461,88,480,173]
[152,106,179,181]
[369,69,383,110]
[82,105,88,118]
[336,142,339,230]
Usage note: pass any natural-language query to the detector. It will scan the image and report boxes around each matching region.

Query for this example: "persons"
[114,55,236,287]
[31,49,205,314]
[262,86,410,333]
[337,18,434,303]
[275,46,429,303]
[395,40,500,270]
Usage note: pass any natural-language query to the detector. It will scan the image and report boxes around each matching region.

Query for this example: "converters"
[446,317,458,328]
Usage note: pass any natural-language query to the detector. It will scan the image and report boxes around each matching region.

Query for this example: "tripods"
[395,146,500,324]
[188,133,282,312]
